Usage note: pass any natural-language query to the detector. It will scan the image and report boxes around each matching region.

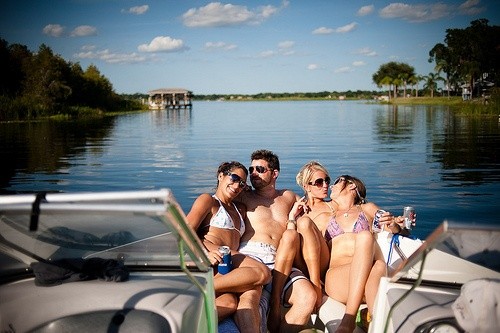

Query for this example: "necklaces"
[340,206,353,217]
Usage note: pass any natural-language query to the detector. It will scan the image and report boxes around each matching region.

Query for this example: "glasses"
[334,177,352,185]
[308,177,330,187]
[249,166,273,173]
[225,171,247,189]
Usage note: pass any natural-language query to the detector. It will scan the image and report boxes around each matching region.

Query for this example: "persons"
[234,150,317,333]
[184,161,272,324]
[268,161,416,333]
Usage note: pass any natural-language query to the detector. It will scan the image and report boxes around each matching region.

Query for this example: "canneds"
[372,209,386,232]
[218,246,230,255]
[404,206,415,229]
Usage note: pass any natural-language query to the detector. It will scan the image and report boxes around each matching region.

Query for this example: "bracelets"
[287,220,296,224]
